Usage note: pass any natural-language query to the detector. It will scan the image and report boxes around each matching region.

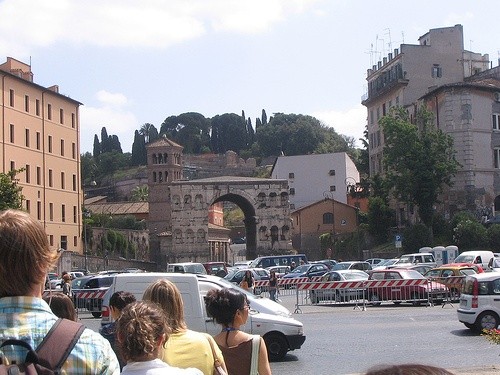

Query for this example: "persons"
[241,271,256,295]
[142,280,227,375]
[43,270,78,322]
[204,289,271,375]
[285,259,309,275]
[215,266,227,278]
[117,300,205,375]
[99,291,135,372]
[0,210,120,375]
[269,270,280,302]
[338,259,342,263]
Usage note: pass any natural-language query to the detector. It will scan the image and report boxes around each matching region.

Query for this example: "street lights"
[346,182,367,261]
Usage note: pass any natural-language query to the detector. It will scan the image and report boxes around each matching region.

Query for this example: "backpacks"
[0,318,87,375]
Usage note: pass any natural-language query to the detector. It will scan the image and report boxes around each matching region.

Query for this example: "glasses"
[244,304,251,310]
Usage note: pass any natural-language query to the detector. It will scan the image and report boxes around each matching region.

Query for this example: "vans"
[99,272,306,363]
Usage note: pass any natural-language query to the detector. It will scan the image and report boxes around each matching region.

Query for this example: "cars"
[232,238,246,244]
[456,272,500,335]
[44,251,500,319]
